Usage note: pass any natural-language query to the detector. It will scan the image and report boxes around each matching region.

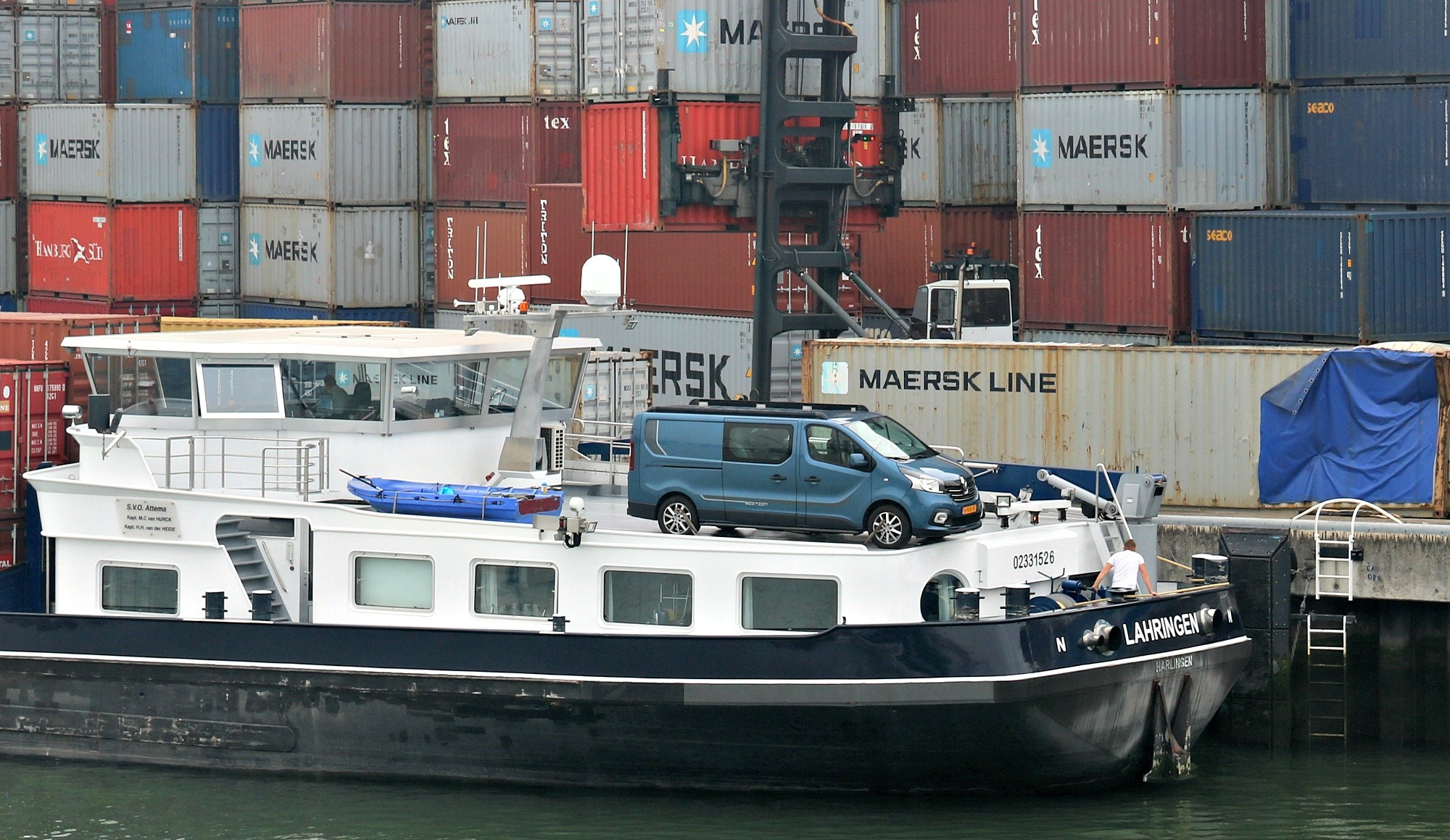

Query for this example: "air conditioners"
[540,426,565,470]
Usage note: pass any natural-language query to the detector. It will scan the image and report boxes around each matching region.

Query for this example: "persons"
[1091,539,1160,598]
[965,300,981,323]
[320,375,350,420]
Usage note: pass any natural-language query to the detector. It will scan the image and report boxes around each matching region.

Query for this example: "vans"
[625,393,983,551]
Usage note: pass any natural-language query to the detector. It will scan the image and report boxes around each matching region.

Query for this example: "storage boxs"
[0,0,1450,571]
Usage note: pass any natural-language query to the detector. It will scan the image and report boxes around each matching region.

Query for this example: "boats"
[338,468,565,525]
[0,251,1259,805]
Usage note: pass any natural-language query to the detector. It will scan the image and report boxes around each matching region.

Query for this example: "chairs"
[346,382,371,415]
